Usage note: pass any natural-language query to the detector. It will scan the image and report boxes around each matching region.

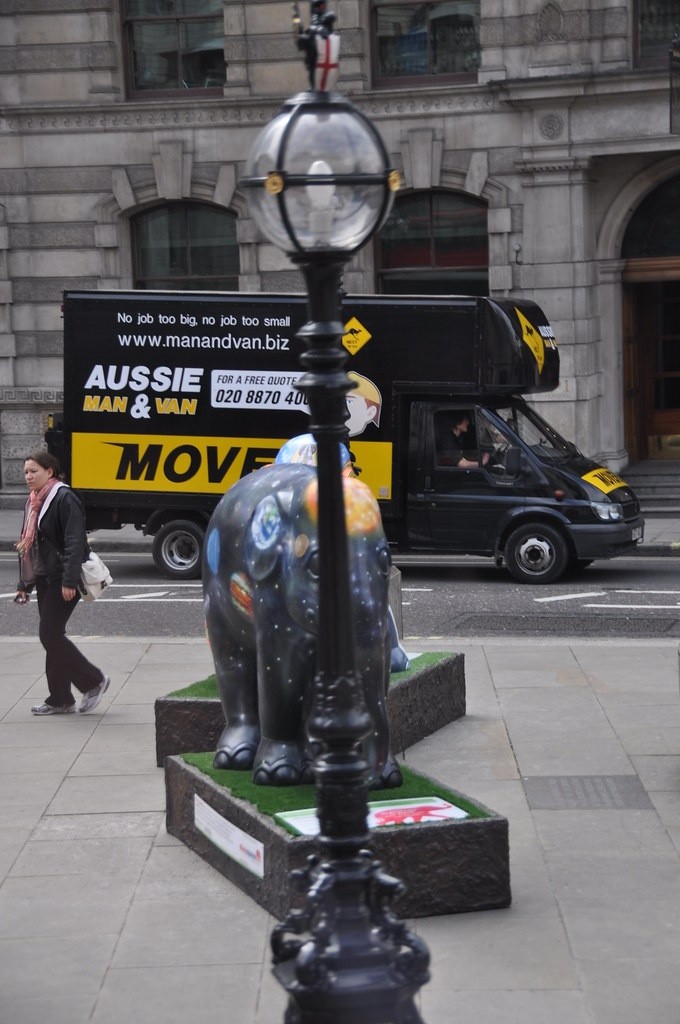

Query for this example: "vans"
[49,289,648,586]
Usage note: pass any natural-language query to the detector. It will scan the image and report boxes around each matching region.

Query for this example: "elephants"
[202,432,411,789]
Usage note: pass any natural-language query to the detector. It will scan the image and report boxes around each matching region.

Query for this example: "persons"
[13,451,111,716]
[437,411,490,467]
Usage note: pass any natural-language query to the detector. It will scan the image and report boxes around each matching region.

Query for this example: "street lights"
[249,0,445,1024]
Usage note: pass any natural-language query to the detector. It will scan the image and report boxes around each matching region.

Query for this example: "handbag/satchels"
[75,552,112,604]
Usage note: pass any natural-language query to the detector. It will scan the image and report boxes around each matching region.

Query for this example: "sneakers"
[30,698,76,715]
[79,674,110,713]
